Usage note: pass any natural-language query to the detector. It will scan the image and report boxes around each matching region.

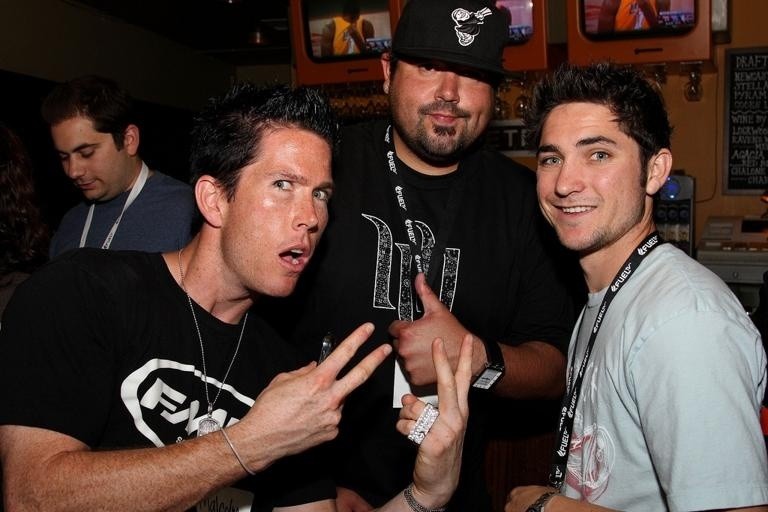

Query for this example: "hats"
[392,1,522,78]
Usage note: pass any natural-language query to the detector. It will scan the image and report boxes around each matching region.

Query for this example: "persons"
[26,85,206,275]
[596,1,660,32]
[507,60,768,512]
[0,87,473,510]
[317,12,377,56]
[322,1,587,510]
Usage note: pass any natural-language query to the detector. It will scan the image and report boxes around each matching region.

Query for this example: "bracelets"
[216,426,258,477]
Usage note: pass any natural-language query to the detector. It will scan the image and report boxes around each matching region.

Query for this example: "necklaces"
[175,247,249,436]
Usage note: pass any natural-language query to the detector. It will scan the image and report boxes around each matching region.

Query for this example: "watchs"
[474,332,505,391]
[529,491,558,506]
